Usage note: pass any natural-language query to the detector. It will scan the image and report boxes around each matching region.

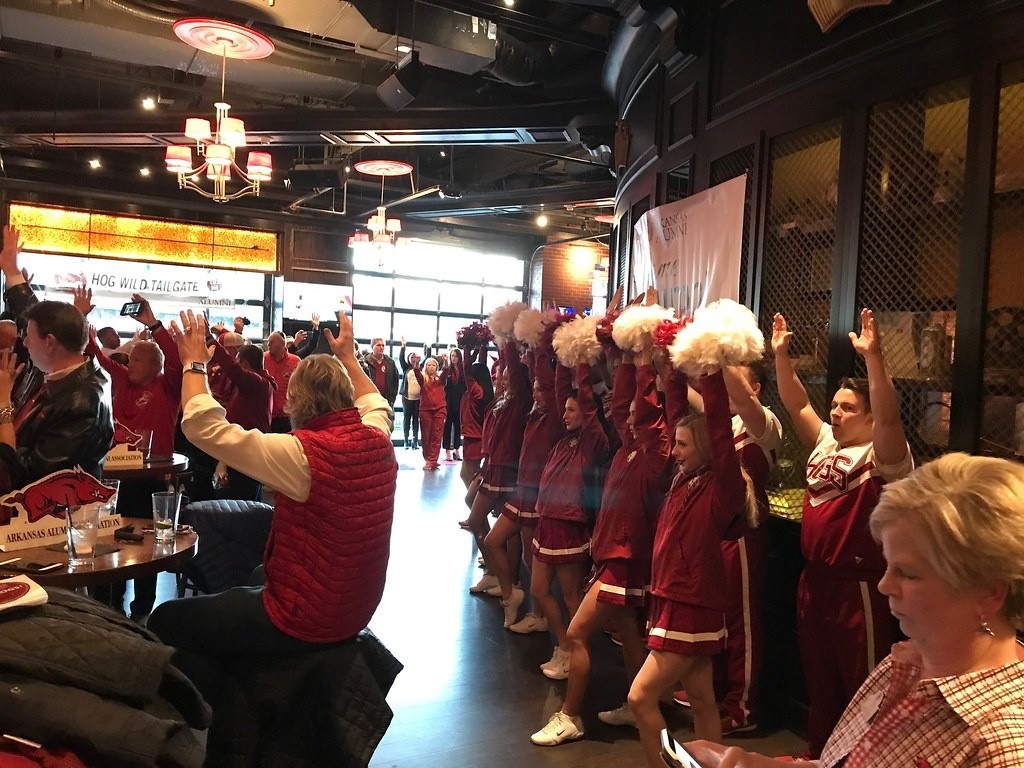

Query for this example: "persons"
[442,349,480,461]
[0,224,112,482]
[460,347,527,596]
[67,284,182,620]
[627,370,758,767]
[399,334,432,449]
[772,310,915,759]
[483,350,565,633]
[672,362,781,739]
[678,454,1024,768]
[145,310,399,656]
[531,283,670,748]
[365,337,398,406]
[413,352,448,470]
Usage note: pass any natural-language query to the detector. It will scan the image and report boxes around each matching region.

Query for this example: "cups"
[263,339,269,351]
[65,504,100,565]
[152,491,182,544]
[135,429,153,459]
[98,479,120,518]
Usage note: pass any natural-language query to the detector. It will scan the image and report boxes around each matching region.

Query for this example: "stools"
[205,625,406,768]
[175,497,274,598]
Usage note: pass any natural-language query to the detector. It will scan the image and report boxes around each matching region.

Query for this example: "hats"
[216,331,244,348]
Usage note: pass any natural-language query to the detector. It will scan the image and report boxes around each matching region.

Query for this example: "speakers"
[376,60,432,111]
[288,163,348,188]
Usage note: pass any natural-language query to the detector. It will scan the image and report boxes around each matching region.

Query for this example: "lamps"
[347,157,416,254]
[535,211,552,229]
[163,14,278,207]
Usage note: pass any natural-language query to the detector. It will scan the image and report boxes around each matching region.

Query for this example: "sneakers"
[719,713,757,735]
[531,710,585,745]
[673,688,692,707]
[540,646,572,680]
[509,611,549,633]
[600,700,640,729]
[774,750,811,764]
[470,574,500,592]
[488,581,521,596]
[500,588,524,628]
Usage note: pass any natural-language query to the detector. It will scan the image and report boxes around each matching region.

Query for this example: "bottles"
[244,338,252,345]
[945,336,952,364]
[918,322,944,378]
[934,147,961,203]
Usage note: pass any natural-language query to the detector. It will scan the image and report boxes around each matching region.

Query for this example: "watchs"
[182,361,206,374]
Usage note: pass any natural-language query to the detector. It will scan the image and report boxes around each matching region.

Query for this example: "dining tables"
[0,513,202,613]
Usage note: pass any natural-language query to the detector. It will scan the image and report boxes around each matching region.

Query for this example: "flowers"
[667,294,767,377]
[455,320,493,345]
[512,308,549,349]
[485,300,528,344]
[551,310,605,367]
[612,303,677,354]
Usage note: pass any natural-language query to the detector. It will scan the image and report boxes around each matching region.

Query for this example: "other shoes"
[612,629,649,647]
[404,444,410,450]
[601,619,617,633]
[411,443,419,449]
[459,518,471,529]
[453,453,463,461]
[424,463,434,470]
[478,555,485,566]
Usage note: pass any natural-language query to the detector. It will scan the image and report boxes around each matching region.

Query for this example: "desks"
[100,450,190,485]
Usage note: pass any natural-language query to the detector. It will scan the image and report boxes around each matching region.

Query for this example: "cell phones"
[142,523,189,531]
[144,457,174,463]
[0,558,63,573]
[659,728,703,768]
[120,302,144,316]
